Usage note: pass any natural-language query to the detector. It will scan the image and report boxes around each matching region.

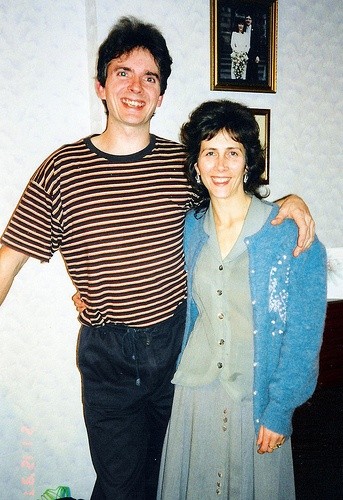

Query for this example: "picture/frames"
[209,0,278,94]
[246,108,271,185]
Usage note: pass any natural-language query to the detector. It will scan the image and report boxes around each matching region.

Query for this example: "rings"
[268,444,275,451]
[277,443,282,448]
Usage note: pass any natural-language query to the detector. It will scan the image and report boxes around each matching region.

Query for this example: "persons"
[0,14,316,500]
[229,14,260,81]
[71,99,328,500]
